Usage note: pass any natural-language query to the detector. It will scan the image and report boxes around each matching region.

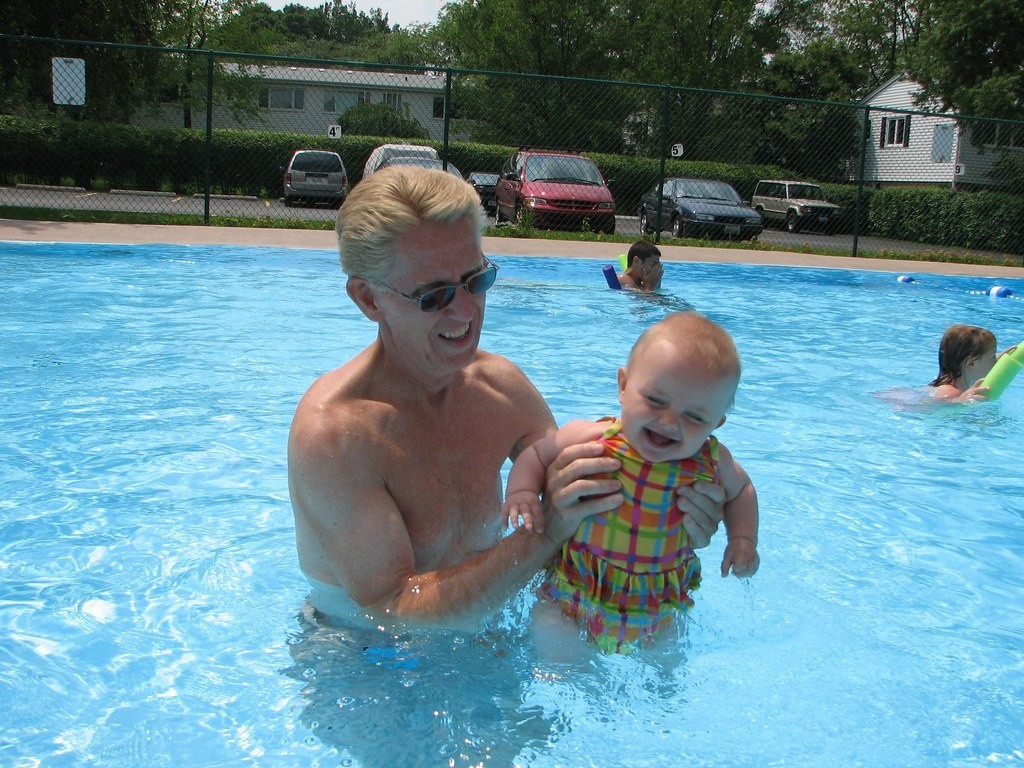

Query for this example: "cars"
[638,179,764,239]
[372,157,467,183]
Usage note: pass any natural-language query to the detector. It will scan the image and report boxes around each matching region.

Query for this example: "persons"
[284,165,730,662]
[912,322,1019,405]
[614,238,665,299]
[494,311,763,679]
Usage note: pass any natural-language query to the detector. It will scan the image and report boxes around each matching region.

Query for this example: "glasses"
[378,254,499,312]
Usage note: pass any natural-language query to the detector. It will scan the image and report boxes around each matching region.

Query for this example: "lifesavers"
[601,252,629,290]
[974,340,1023,403]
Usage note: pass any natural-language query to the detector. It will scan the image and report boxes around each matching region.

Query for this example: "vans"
[496,152,617,236]
[283,150,348,208]
[360,145,440,178]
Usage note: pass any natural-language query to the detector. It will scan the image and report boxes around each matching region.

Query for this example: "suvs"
[467,171,501,208]
[751,180,843,236]
